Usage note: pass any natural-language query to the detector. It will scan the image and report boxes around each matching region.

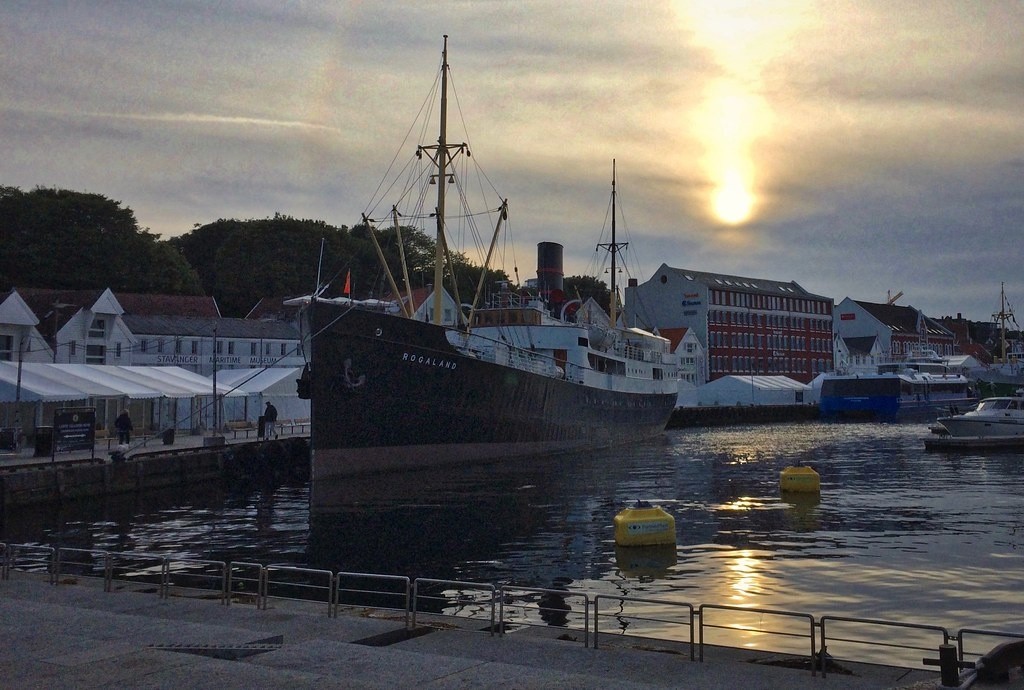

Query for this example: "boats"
[819,375,902,418]
[937,396,1024,438]
[966,280,1024,400]
[291,34,679,481]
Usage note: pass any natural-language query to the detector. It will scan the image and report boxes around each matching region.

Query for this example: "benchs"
[274,419,295,435]
[229,422,255,439]
[294,418,311,434]
[105,428,152,449]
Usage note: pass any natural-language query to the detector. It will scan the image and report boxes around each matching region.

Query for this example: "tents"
[674,379,697,405]
[0,361,250,435]
[941,354,981,374]
[697,374,812,405]
[807,372,829,402]
[209,367,311,422]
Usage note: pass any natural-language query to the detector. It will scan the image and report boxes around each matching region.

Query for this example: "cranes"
[886,289,904,306]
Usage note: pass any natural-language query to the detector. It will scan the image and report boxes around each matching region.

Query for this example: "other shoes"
[266,438,269,440]
[275,434,278,439]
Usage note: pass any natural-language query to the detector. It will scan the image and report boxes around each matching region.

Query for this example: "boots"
[118,444,129,451]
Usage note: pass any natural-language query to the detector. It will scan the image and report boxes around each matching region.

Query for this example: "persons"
[114,409,133,445]
[263,401,279,440]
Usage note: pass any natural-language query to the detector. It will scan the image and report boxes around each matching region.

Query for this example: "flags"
[344,271,351,293]
